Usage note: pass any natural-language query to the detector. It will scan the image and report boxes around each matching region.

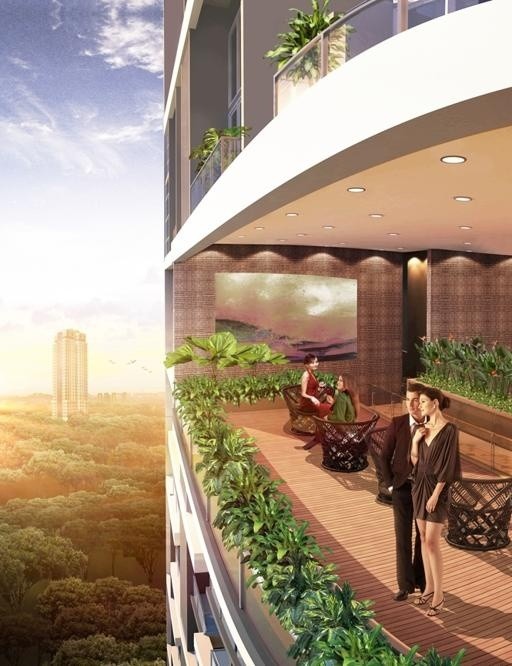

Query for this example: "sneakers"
[413,579,426,593]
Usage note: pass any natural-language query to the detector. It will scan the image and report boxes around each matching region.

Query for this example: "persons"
[299,352,334,419]
[379,383,432,601]
[409,387,461,616]
[326,372,361,435]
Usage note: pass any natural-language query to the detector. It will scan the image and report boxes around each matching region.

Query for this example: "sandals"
[413,590,435,605]
[426,596,446,616]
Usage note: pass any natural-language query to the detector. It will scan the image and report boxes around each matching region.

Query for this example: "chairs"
[285,384,512,551]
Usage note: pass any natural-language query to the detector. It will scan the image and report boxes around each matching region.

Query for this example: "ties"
[412,422,420,426]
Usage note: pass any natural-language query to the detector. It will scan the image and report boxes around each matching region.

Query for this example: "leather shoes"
[393,585,416,601]
